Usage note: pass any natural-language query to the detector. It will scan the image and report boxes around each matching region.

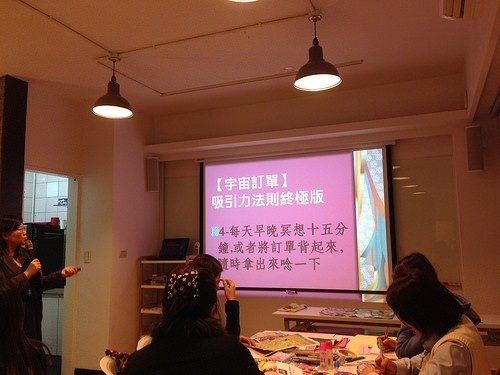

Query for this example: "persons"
[194,253,241,341]
[0,214,82,375]
[374,273,492,375]
[377,251,481,359]
[124,263,264,375]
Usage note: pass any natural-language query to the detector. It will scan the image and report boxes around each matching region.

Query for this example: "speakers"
[146,157,159,192]
[455,125,485,172]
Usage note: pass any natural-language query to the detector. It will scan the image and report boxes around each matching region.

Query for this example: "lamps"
[92,52,134,119]
[293,15,343,92]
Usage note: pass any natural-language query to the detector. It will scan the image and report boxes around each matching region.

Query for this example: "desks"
[273,303,500,343]
[246,330,399,375]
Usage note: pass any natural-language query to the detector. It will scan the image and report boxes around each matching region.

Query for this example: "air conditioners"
[438,0,467,21]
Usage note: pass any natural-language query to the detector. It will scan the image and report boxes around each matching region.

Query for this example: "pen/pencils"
[378,337,386,375]
[385,325,389,340]
[348,356,365,362]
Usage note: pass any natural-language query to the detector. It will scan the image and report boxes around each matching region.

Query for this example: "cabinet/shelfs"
[40,290,64,366]
[139,259,194,313]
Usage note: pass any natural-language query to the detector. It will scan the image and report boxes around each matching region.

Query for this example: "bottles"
[320,343,325,371]
[325,341,333,371]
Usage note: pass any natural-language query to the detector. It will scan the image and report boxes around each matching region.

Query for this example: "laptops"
[144,237,190,260]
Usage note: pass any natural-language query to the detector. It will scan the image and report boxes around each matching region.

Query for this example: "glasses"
[18,227,26,233]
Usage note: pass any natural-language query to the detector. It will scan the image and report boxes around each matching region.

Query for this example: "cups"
[383,296,395,318]
[51,218,59,226]
[60,220,67,229]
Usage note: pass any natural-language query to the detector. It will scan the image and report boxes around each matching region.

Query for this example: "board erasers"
[285,289,298,294]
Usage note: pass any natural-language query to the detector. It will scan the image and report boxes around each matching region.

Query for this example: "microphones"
[25,240,35,260]
